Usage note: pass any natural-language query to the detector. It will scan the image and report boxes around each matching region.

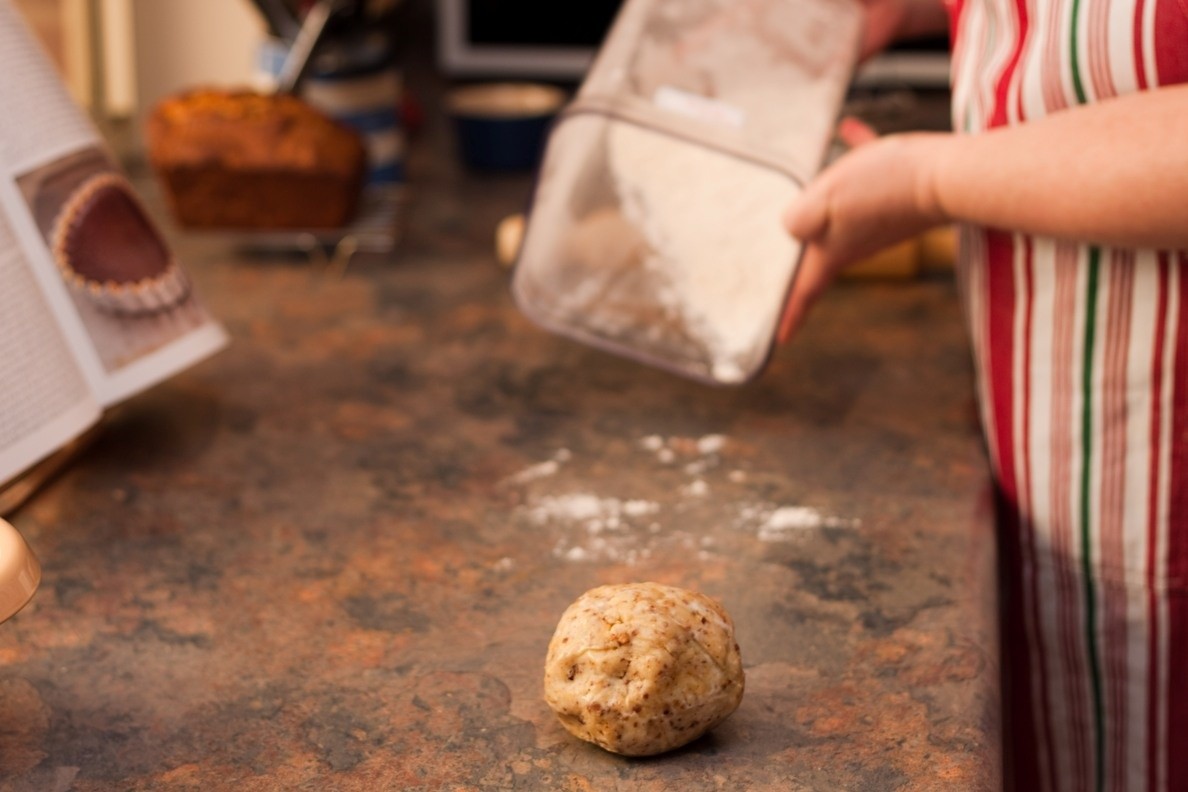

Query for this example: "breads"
[147,84,366,238]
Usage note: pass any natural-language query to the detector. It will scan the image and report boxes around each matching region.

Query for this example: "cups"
[448,82,561,175]
[270,29,407,182]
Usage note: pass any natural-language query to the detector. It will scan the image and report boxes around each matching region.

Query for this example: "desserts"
[52,173,190,316]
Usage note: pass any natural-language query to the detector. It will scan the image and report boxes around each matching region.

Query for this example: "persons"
[773,0,1188,792]
